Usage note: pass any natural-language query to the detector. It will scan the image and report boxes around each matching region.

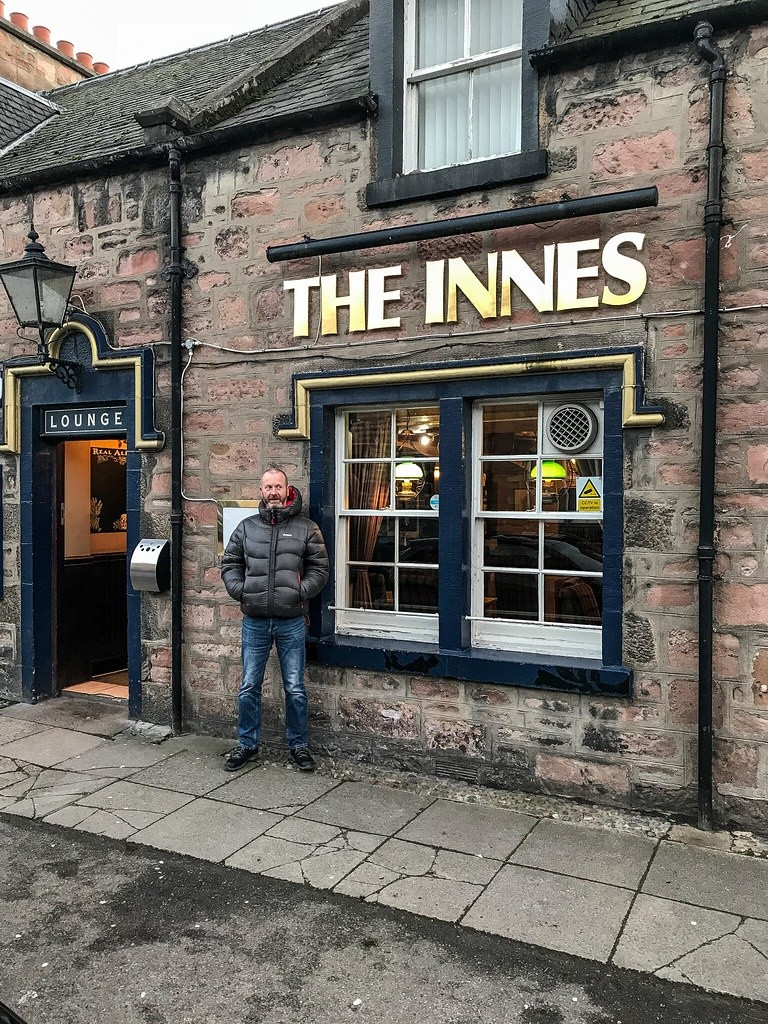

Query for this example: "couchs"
[386,538,440,615]
[493,533,602,626]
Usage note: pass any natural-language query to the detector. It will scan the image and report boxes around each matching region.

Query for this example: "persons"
[221,468,330,770]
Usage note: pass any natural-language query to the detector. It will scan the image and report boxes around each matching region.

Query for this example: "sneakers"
[289,743,316,771]
[224,748,259,771]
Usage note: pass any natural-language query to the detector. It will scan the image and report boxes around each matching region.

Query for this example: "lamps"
[0,221,84,398]
[395,428,425,501]
[530,461,566,487]
